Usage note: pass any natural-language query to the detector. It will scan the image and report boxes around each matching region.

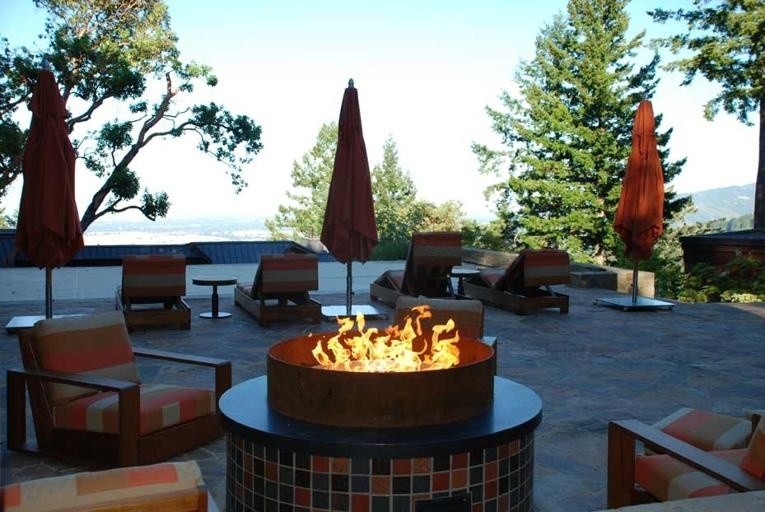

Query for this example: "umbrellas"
[612,93,664,303]
[15,60,85,321]
[321,79,378,312]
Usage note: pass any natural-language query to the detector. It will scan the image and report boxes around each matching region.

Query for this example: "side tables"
[451,269,480,295]
[192,278,237,319]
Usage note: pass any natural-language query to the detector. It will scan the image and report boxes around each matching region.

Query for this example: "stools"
[644,407,752,455]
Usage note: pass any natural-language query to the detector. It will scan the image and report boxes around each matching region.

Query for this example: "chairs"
[7,310,231,468]
[0,461,220,512]
[370,232,462,309]
[462,250,569,315]
[394,296,497,376]
[607,409,765,509]
[115,254,191,333]
[234,253,321,326]
